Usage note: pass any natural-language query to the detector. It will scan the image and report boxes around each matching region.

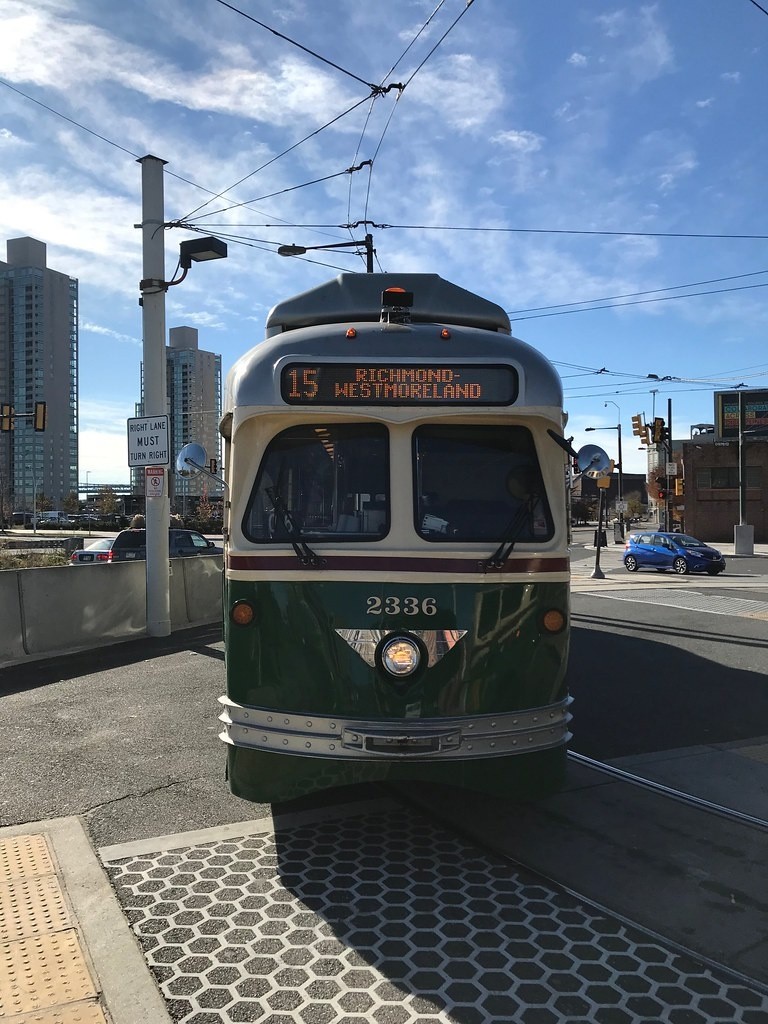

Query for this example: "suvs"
[107,528,223,560]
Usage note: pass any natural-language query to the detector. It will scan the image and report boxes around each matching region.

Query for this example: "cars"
[39,517,69,523]
[71,540,116,564]
[76,516,100,522]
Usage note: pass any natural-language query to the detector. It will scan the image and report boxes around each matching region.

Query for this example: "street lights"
[605,401,622,507]
[138,238,228,636]
[585,424,625,544]
[277,234,375,274]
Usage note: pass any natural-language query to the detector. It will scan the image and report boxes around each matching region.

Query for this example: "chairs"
[451,500,528,538]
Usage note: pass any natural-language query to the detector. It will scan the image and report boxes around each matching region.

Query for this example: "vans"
[622,532,726,575]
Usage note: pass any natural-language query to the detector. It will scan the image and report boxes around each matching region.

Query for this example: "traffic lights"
[0,404,15,432]
[633,413,642,436]
[652,417,664,443]
[640,426,650,445]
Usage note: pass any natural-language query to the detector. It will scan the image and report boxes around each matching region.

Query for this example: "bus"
[177,271,610,802]
